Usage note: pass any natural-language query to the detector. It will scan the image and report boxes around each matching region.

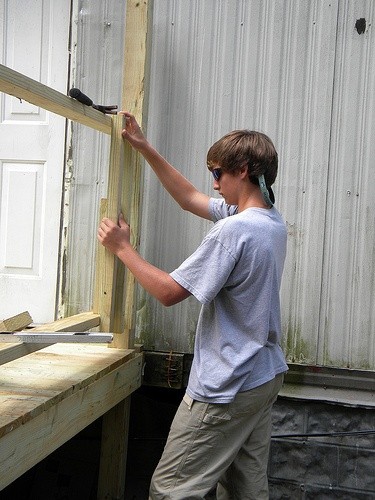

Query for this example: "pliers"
[68,87,118,116]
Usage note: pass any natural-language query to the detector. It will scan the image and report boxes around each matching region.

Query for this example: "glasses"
[213,167,224,181]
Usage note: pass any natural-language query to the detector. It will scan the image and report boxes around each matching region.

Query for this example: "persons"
[97,111,288,499]
